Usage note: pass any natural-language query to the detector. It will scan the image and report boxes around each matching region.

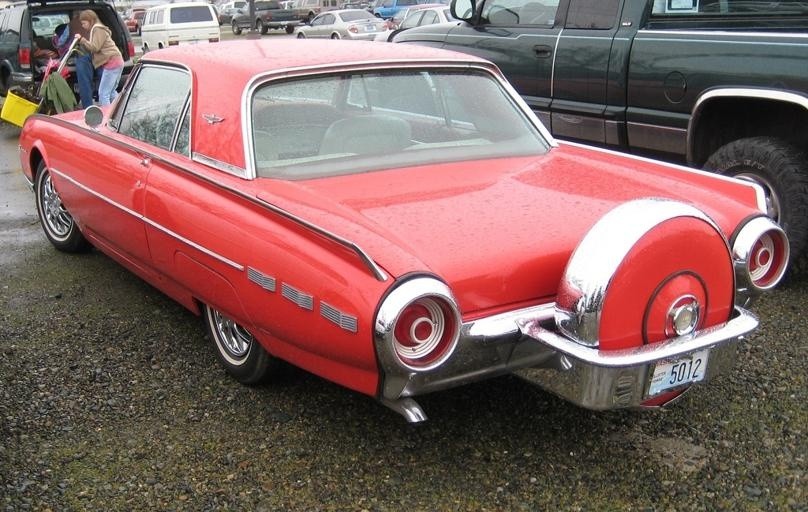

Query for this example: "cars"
[212,0,249,26]
[18,38,792,425]
[279,0,449,20]
[388,0,808,270]
[398,7,456,30]
[118,6,146,36]
[295,9,389,41]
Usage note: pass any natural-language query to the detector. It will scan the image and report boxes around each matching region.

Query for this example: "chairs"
[318,113,412,164]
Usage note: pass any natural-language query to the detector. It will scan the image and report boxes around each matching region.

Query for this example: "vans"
[141,2,221,53]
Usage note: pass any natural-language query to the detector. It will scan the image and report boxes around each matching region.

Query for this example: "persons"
[74,8,125,107]
[56,8,104,109]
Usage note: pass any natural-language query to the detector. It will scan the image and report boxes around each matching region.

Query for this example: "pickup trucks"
[231,0,313,35]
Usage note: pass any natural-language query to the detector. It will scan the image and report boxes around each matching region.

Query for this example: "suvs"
[0,0,136,100]
